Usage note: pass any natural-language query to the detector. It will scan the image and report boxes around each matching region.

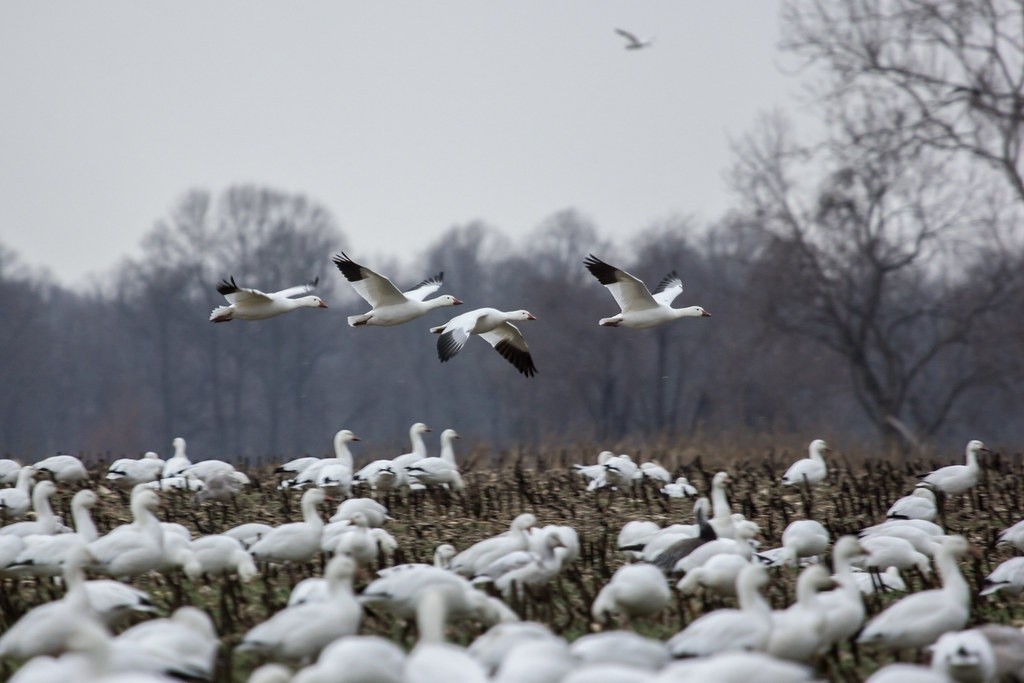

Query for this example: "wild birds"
[208,249,715,379]
[613,26,654,52]
[1,418,1023,683]
